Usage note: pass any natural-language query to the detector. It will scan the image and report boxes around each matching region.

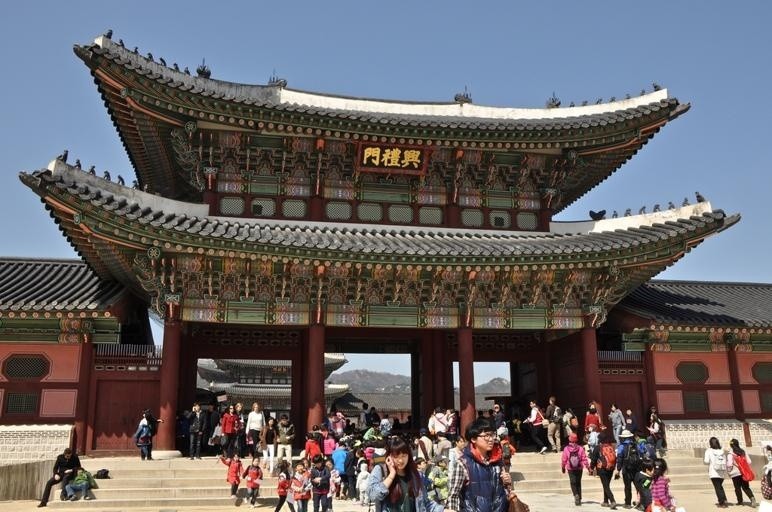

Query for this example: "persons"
[175,396,676,512]
[726,439,756,508]
[762,444,772,488]
[37,447,81,507]
[133,409,164,461]
[65,468,98,501]
[703,436,728,508]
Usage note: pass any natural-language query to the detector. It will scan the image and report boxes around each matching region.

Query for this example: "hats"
[618,430,633,437]
[569,433,577,442]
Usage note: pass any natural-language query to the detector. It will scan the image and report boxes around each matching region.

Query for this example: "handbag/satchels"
[659,422,665,435]
[542,418,549,429]
[569,451,581,468]
[506,491,530,512]
[502,443,511,459]
[231,435,240,450]
[645,443,656,460]
[96,469,109,479]
[220,436,228,446]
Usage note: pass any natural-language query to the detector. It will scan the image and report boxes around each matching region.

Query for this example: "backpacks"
[732,454,754,481]
[600,444,616,471]
[621,442,640,472]
[713,455,727,471]
[552,405,563,423]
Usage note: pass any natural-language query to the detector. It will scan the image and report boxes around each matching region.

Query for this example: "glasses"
[477,432,497,441]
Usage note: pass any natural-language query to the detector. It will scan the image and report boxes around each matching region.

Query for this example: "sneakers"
[539,446,548,454]
[571,418,579,428]
